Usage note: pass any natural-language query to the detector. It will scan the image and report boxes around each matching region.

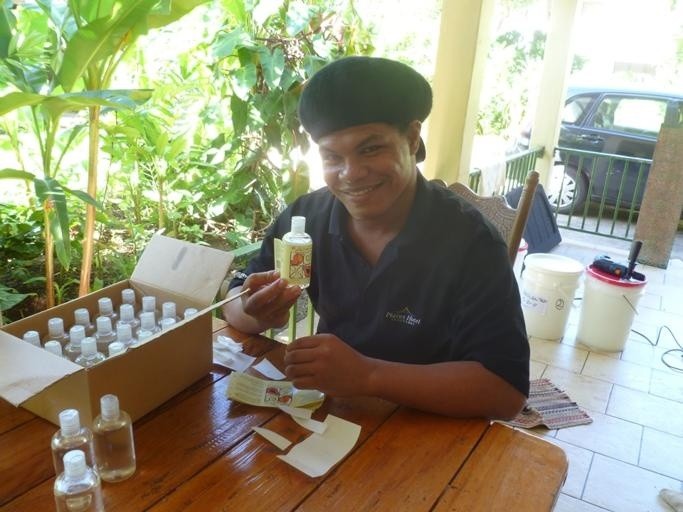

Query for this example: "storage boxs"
[0,235,234,436]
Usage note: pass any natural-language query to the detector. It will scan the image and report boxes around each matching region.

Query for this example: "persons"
[219,52,534,427]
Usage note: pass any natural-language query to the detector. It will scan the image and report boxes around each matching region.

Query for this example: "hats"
[299,57,432,164]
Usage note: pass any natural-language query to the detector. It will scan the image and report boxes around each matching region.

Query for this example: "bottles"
[53,449,104,512]
[49,409,96,476]
[93,394,138,483]
[23,287,199,367]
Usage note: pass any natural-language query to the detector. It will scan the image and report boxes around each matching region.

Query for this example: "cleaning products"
[23,287,200,367]
[49,392,139,512]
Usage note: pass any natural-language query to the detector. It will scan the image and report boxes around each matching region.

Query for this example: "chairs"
[428,163,540,268]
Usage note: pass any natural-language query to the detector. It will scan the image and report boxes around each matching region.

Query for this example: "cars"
[508,86,682,236]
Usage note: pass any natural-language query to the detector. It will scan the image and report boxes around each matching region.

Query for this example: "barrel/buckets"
[576,263,648,354]
[520,253,584,340]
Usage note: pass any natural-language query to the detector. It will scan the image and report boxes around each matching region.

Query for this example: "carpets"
[491,377,592,428]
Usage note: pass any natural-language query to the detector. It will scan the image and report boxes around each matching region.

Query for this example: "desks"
[2,313,568,510]
